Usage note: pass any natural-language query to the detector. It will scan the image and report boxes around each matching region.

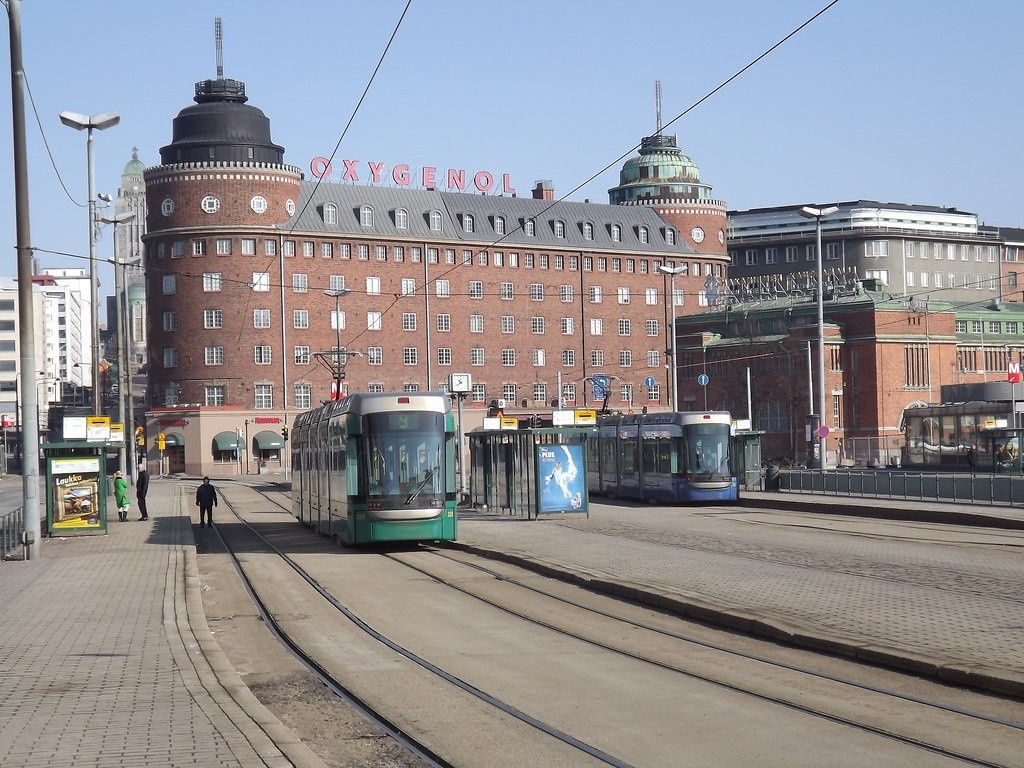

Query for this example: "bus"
[488,407,740,507]
[290,390,458,548]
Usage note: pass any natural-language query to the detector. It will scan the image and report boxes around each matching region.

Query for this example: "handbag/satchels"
[124,493,129,498]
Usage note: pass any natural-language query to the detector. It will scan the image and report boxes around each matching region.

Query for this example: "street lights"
[74,362,95,415]
[60,110,120,418]
[99,211,137,483]
[107,255,142,486]
[799,206,840,470]
[37,381,56,468]
[15,370,45,469]
[659,264,689,413]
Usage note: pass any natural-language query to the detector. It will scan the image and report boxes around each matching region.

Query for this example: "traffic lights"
[282,427,288,441]
[136,433,140,442]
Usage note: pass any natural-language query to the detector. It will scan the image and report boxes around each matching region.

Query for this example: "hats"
[137,464,145,472]
[116,471,122,476]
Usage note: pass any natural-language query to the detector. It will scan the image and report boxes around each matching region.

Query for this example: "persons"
[547,461,575,497]
[113,471,131,522]
[196,476,217,528]
[966,445,977,479]
[136,464,150,521]
[996,440,1018,466]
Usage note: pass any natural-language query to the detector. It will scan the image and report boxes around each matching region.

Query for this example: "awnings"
[253,430,285,450]
[164,433,184,446]
[213,432,246,451]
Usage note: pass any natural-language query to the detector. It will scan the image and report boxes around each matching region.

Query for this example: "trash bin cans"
[765,465,780,493]
[107,477,115,496]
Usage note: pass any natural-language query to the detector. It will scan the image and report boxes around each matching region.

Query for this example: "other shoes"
[209,524,212,528]
[138,517,148,521]
[201,524,205,527]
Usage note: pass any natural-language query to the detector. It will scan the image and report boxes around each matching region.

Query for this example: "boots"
[123,511,129,521]
[118,512,123,522]
